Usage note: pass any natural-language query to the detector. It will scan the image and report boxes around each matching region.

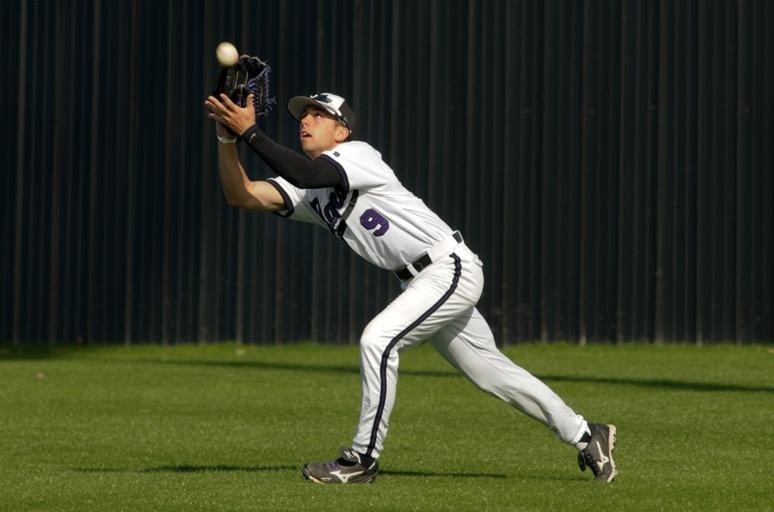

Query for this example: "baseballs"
[217,42,239,66]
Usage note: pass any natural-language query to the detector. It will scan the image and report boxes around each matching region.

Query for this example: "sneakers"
[303,447,379,484]
[578,423,616,484]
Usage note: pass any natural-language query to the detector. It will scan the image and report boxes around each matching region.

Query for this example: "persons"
[202,91,617,487]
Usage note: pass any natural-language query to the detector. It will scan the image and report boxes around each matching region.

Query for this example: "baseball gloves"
[220,55,278,137]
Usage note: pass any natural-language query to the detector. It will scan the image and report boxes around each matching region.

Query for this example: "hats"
[287,92,354,142]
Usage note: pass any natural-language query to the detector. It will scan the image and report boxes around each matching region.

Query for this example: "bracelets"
[216,132,238,146]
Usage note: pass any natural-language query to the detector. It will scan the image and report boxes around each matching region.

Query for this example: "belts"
[395,231,462,281]
